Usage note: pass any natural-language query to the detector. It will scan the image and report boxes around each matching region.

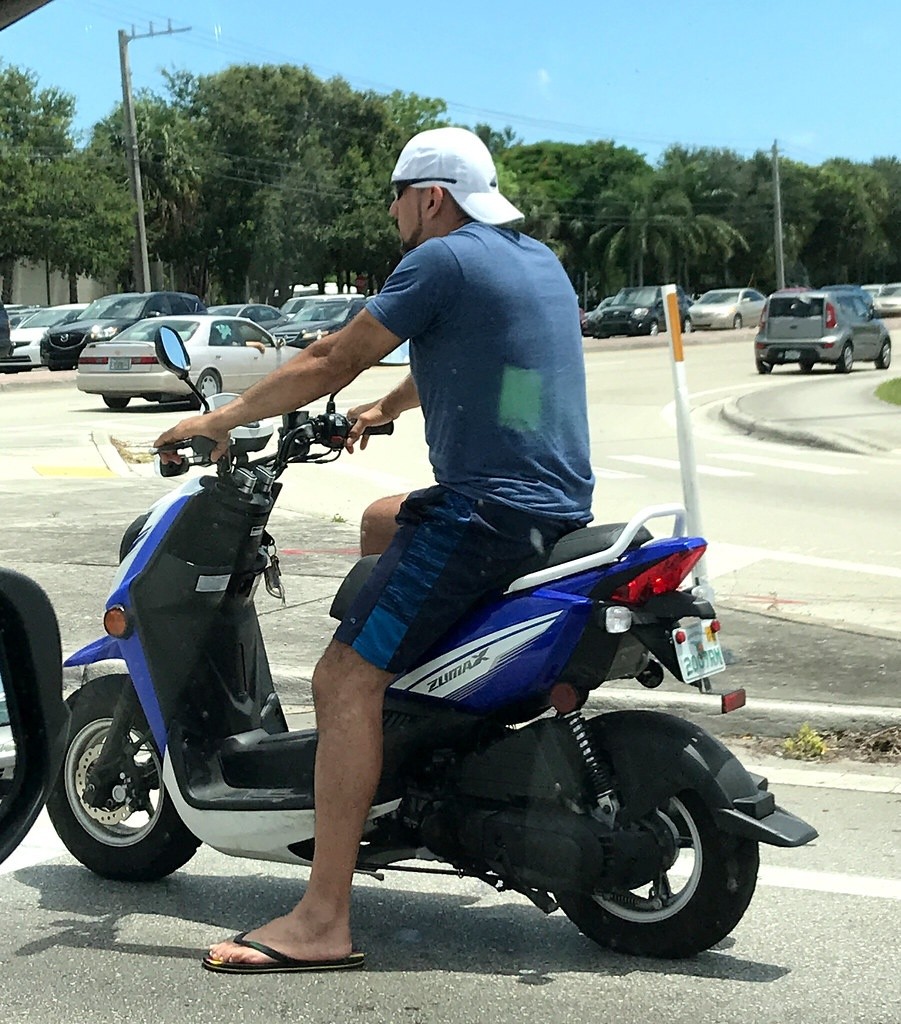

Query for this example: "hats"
[390,127,527,227]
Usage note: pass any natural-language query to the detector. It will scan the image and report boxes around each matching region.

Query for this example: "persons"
[150,127,597,976]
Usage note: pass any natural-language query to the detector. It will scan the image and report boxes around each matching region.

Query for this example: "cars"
[73,315,315,411]
[3,304,43,333]
[580,295,632,338]
[862,283,901,313]
[687,288,768,332]
[595,285,693,337]
[0,303,120,366]
[185,304,292,344]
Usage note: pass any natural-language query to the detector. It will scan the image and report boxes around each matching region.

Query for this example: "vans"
[281,294,365,321]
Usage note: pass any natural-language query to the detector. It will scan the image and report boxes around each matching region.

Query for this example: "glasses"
[390,178,457,201]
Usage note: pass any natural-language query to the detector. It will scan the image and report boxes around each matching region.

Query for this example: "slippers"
[201,927,366,975]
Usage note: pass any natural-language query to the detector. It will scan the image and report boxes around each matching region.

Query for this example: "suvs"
[40,291,209,371]
[752,286,892,374]
[261,298,368,350]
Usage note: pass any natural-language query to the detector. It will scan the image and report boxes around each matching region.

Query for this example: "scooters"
[45,325,820,961]
